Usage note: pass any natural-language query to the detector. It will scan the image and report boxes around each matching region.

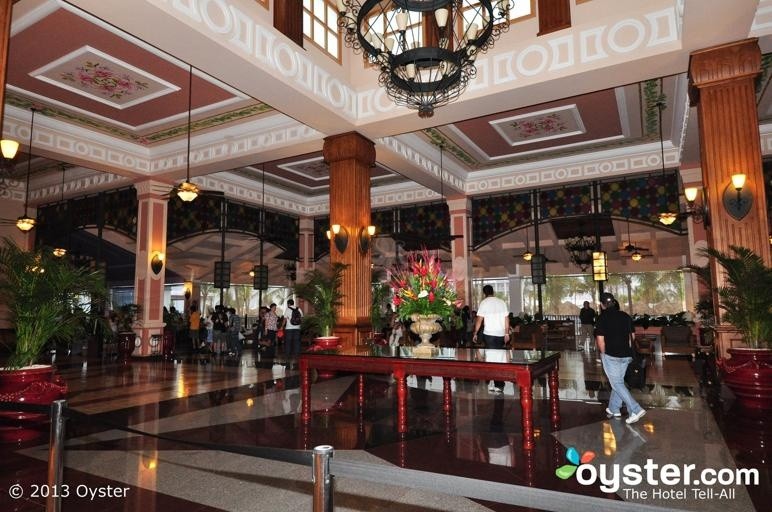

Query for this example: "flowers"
[384,243,465,329]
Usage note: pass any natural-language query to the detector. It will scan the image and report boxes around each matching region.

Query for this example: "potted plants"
[287,260,352,380]
[370,279,389,345]
[0,234,184,443]
[677,243,772,410]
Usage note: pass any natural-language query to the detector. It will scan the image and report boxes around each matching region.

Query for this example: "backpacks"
[288,306,302,325]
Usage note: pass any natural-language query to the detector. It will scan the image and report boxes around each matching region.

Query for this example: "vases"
[407,314,443,356]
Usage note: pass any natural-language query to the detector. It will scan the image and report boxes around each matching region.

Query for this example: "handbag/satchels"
[625,358,647,389]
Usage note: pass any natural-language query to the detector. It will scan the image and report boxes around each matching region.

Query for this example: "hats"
[600,292,615,304]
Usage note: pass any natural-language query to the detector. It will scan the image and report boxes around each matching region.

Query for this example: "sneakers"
[605,408,621,417]
[489,384,504,392]
[625,409,647,424]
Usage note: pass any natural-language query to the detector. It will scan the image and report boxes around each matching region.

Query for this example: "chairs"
[636,339,654,367]
[512,327,546,351]
[663,327,695,359]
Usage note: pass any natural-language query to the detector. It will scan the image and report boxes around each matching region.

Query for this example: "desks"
[295,345,561,450]
[634,327,663,361]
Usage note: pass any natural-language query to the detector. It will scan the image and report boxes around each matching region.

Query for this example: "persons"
[163,299,304,358]
[449,300,597,350]
[472,284,510,392]
[385,304,393,341]
[596,293,647,425]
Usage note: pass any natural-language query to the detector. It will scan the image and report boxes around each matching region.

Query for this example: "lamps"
[359,226,376,256]
[337,0,514,118]
[332,224,349,253]
[0,66,269,291]
[524,102,754,284]
